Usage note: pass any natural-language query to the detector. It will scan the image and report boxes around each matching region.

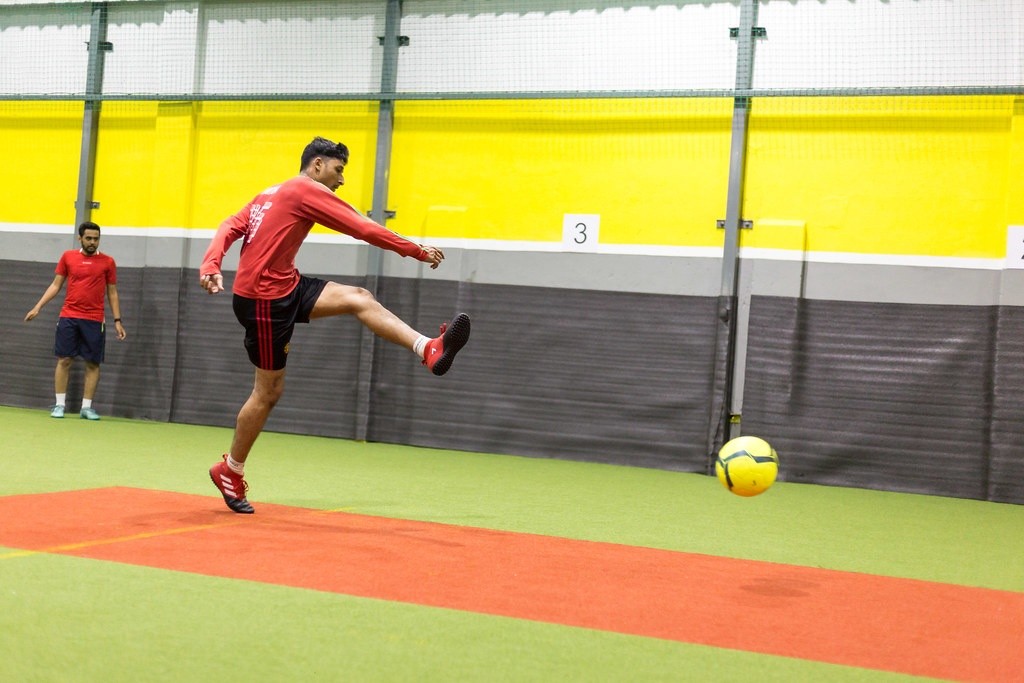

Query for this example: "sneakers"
[48,404,65,418]
[421,313,471,376]
[209,454,255,513]
[80,406,100,420]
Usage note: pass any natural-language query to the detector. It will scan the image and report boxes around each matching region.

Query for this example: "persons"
[199,137,470,513]
[23,221,126,419]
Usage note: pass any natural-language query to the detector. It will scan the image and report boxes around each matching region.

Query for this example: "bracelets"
[115,318,122,323]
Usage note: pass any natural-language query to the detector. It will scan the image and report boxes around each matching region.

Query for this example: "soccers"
[715,436,779,497]
